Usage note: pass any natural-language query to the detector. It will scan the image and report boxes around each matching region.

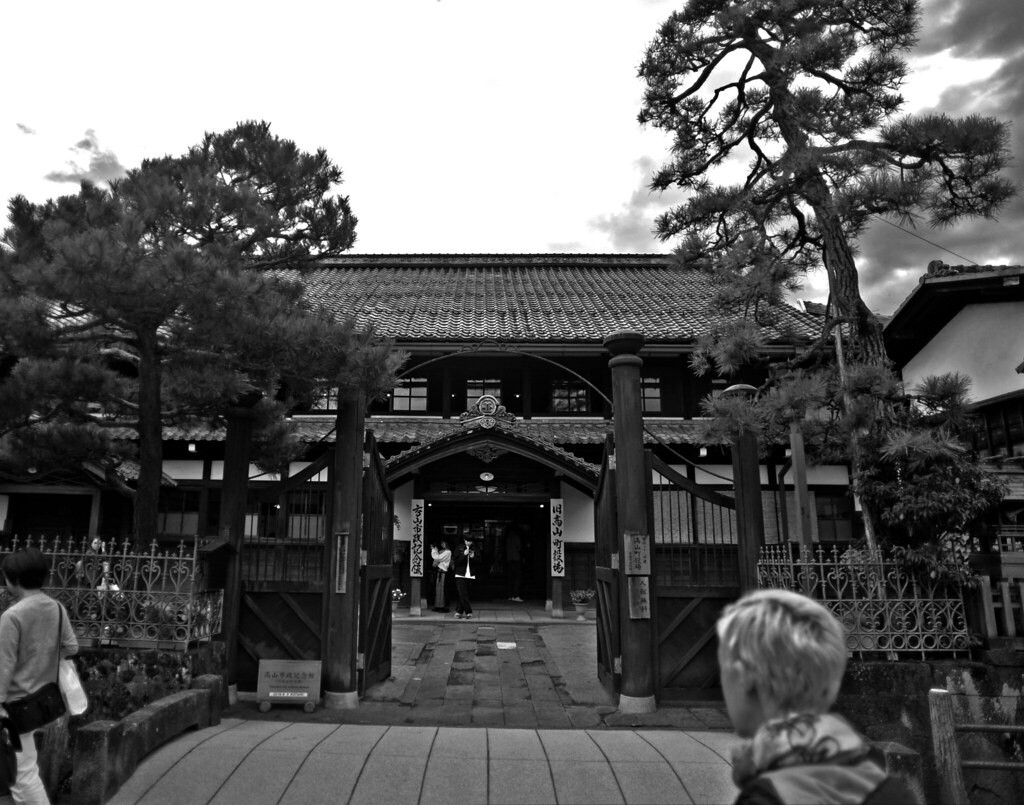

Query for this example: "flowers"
[391,589,406,601]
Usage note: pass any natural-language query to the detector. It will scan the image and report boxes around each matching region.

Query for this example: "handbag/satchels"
[58,660,87,716]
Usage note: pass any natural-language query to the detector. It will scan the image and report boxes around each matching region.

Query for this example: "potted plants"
[570,589,596,621]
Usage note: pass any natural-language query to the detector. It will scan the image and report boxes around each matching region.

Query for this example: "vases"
[392,601,400,619]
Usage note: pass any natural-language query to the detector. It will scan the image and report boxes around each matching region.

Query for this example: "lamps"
[480,472,494,481]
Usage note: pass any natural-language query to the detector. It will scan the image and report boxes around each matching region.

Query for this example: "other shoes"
[508,597,512,601]
[440,607,449,613]
[512,597,523,602]
[466,614,473,619]
[454,613,462,618]
[432,607,440,612]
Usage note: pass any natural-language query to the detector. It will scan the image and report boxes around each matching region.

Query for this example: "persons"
[506,529,523,602]
[430,538,452,612]
[0,548,79,805]
[78,536,108,615]
[453,532,478,618]
[715,589,926,804]
